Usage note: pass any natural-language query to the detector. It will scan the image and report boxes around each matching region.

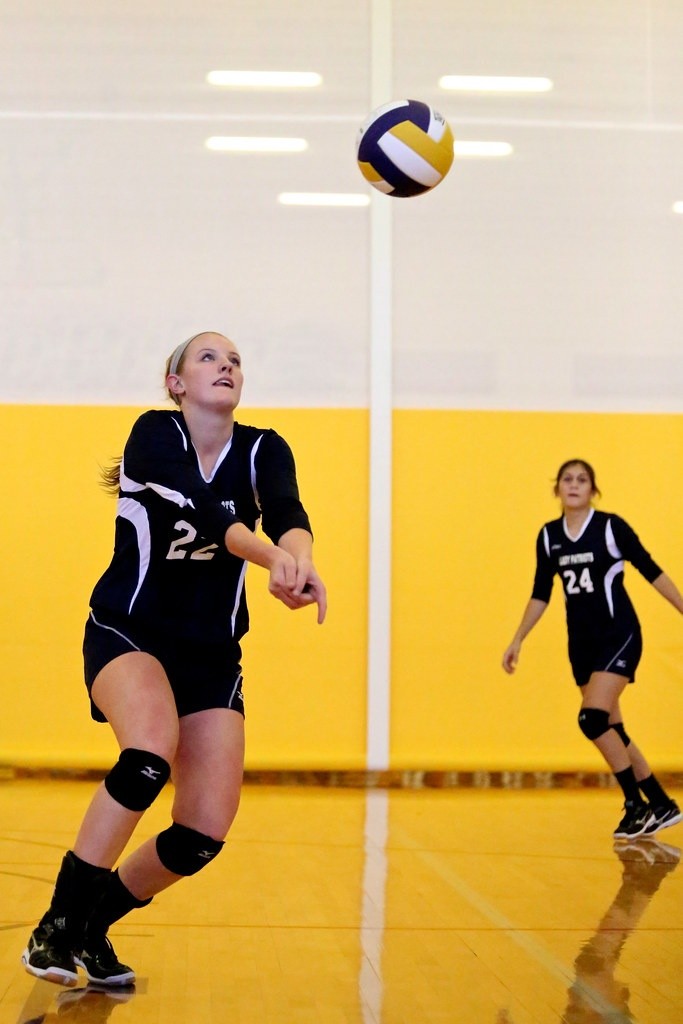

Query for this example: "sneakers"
[75,934,137,987]
[21,911,79,987]
[644,801,681,836]
[614,801,645,840]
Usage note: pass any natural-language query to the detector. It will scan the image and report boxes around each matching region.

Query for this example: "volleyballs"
[354,98,454,198]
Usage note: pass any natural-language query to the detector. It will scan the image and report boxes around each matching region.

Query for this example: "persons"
[22,331,328,986]
[503,459,683,840]
